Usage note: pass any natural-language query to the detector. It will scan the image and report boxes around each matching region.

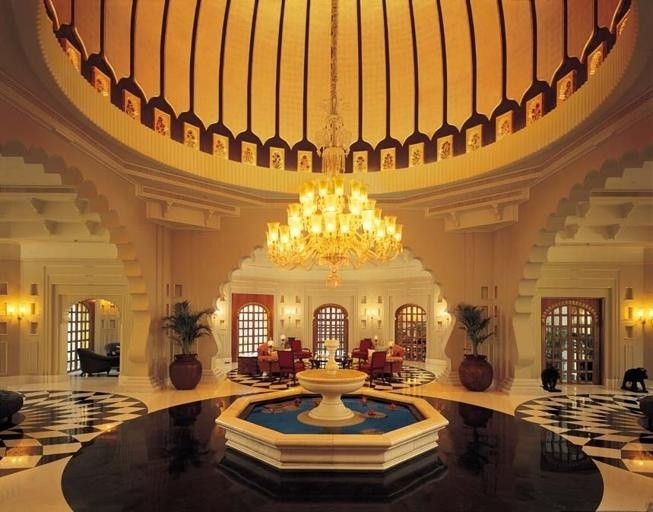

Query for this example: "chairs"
[256,337,312,385]
[75,342,119,374]
[350,337,404,387]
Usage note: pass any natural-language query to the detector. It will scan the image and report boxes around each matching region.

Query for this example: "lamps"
[263,2,406,291]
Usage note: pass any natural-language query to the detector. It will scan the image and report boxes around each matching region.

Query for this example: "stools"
[0,388,23,425]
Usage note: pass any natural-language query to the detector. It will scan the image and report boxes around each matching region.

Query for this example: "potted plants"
[450,300,495,393]
[156,298,215,389]
